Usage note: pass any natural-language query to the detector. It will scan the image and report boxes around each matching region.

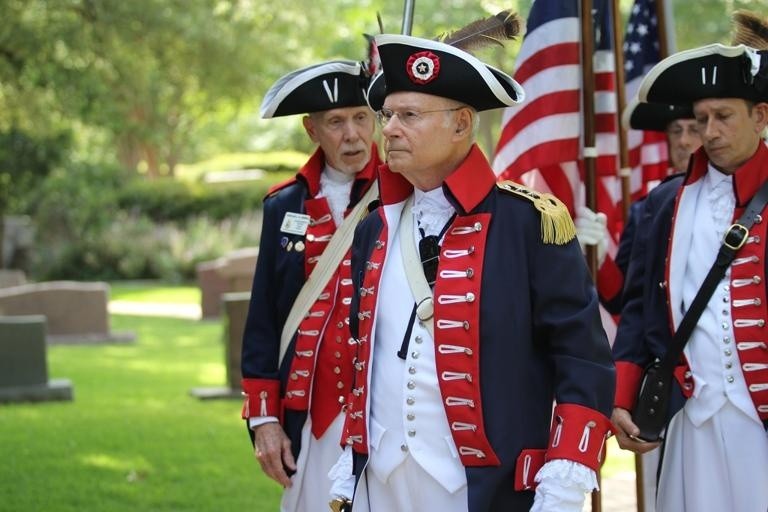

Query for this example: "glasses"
[377,105,466,128]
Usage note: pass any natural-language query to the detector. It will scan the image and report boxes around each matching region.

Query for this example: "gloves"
[329,446,356,501]
[575,207,610,265]
[530,460,599,509]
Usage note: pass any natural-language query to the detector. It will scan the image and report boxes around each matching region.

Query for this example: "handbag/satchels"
[631,358,674,441]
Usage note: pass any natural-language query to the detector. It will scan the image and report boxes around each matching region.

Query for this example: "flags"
[491,1,680,352]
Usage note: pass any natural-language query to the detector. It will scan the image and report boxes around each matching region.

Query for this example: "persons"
[325,32,617,512]
[572,86,702,329]
[238,58,387,512]
[607,43,766,511]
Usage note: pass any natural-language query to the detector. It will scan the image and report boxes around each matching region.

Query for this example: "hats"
[260,61,374,119]
[621,44,766,130]
[367,34,525,111]
